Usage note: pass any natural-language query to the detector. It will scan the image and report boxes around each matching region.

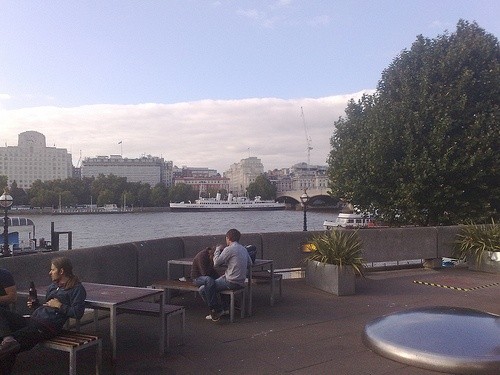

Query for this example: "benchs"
[36,272,285,375]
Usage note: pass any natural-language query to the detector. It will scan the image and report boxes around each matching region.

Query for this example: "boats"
[170,189,286,211]
[323,213,372,229]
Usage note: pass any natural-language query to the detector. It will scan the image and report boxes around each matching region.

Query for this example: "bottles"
[29,281,36,307]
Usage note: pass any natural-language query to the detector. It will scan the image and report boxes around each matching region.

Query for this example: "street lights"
[0,187,14,257]
[299,192,310,232]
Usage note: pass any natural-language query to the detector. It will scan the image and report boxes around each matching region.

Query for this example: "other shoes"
[224,311,229,315]
[0,340,21,355]
[206,310,224,319]
[210,309,220,322]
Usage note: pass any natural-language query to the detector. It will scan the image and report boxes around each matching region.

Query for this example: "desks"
[11,282,166,362]
[167,257,275,318]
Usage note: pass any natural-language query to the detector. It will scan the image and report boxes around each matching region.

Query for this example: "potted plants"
[452,217,500,274]
[306,225,365,295]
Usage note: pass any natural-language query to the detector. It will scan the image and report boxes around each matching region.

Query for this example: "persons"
[0,256,86,375]
[199,229,252,320]
[191,246,221,321]
[0,269,18,343]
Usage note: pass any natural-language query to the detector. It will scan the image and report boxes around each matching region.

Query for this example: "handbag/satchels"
[243,245,256,264]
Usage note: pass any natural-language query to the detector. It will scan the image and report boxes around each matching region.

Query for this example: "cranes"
[300,106,314,165]
